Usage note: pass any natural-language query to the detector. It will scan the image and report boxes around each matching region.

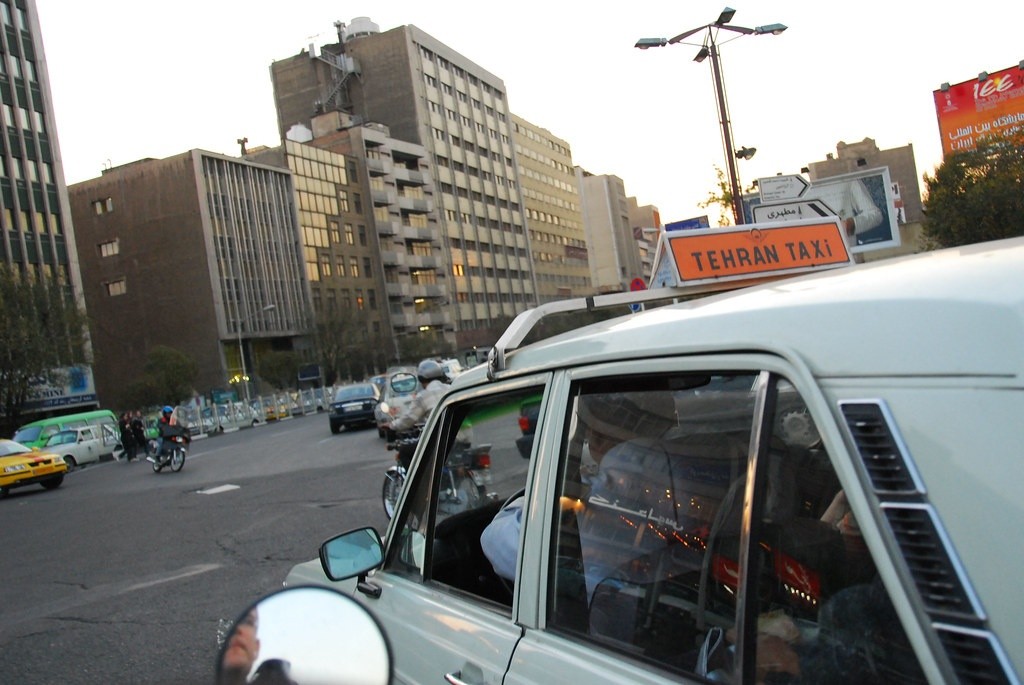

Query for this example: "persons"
[802,178,884,245]
[378,359,473,476]
[114,410,155,464]
[479,390,927,685]
[155,405,181,466]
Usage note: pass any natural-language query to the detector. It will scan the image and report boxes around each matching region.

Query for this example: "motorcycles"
[378,401,499,533]
[147,425,191,473]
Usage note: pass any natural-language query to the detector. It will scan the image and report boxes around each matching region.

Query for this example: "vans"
[39,422,125,473]
[10,409,123,474]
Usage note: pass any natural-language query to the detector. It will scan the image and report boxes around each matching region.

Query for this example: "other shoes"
[116,456,119,462]
[155,456,162,467]
[130,458,139,463]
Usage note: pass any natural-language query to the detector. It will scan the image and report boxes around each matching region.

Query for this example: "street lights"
[238,304,276,399]
[634,6,789,226]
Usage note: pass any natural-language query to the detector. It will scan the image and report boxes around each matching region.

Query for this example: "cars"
[0,437,69,494]
[515,396,542,458]
[187,357,460,444]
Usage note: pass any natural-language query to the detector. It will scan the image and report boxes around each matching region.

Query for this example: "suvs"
[284,215,1024,685]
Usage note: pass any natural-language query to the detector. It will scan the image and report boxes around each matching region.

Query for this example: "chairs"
[579,429,801,665]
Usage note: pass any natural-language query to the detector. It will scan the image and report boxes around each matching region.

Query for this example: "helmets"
[417,361,443,379]
[163,406,173,416]
